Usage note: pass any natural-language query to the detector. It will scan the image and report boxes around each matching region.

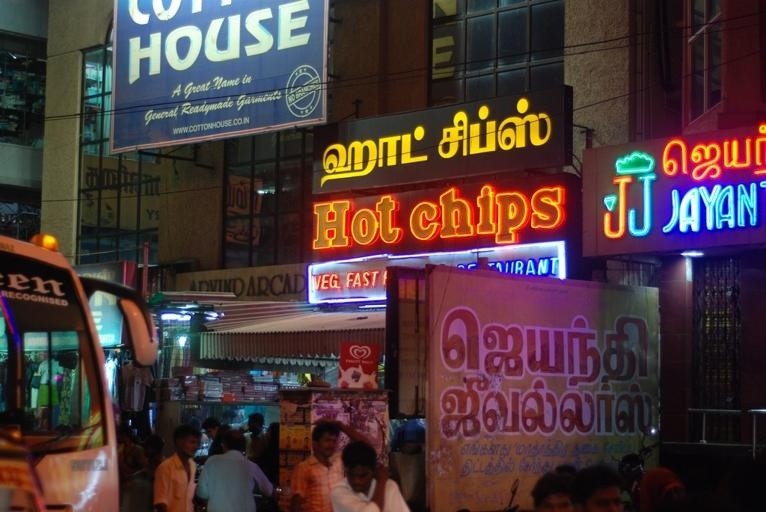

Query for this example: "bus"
[0,233,157,510]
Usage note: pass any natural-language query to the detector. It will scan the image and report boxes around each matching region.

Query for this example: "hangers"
[105,349,136,367]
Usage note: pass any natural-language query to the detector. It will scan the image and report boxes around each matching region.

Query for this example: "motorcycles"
[458,478,520,511]
[610,425,662,512]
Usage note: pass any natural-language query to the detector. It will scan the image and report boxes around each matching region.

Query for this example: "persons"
[201,416,229,455]
[564,464,626,512]
[327,440,411,511]
[634,465,690,511]
[530,462,580,511]
[191,429,277,511]
[243,412,272,466]
[151,423,201,511]
[116,419,155,485]
[285,416,370,512]
